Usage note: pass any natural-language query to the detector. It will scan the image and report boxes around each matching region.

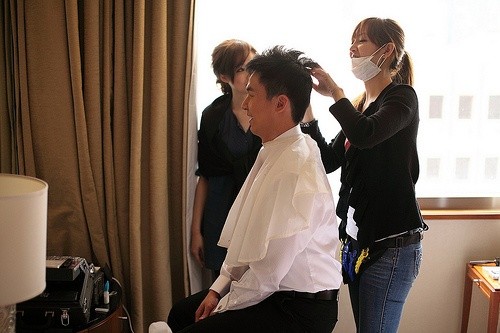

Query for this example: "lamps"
[0,173,48,333]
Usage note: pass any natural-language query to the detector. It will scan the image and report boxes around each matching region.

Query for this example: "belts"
[273,289,337,301]
[379,232,420,248]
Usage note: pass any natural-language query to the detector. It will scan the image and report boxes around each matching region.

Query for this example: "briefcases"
[16,254,105,329]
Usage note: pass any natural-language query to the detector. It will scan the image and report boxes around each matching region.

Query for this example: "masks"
[351,43,388,83]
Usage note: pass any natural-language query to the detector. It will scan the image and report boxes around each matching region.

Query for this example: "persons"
[296,17,428,332]
[190,39,264,287]
[167,44,342,333]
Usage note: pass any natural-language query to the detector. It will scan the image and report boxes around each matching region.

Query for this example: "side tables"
[461,258,500,333]
